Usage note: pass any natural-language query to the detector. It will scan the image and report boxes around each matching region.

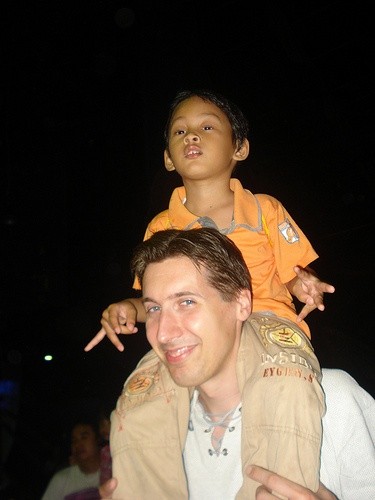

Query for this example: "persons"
[100,225,375,500]
[40,414,102,500]
[93,374,127,482]
[85,85,337,500]
